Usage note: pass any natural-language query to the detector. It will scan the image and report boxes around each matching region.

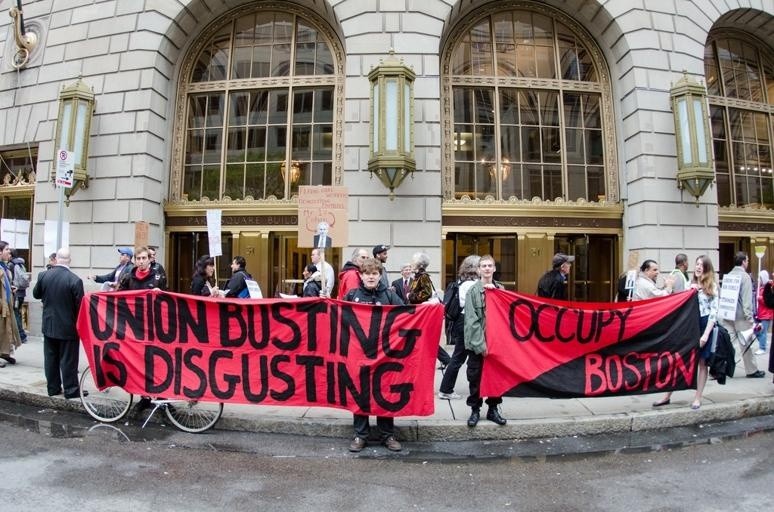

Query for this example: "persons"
[616,248,770,415]
[0,239,33,373]
[82,246,264,409]
[435,253,508,427]
[312,222,332,249]
[37,248,95,401]
[318,257,446,454]
[297,245,450,370]
[539,251,575,306]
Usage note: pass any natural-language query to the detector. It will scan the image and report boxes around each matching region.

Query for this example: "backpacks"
[443,277,466,320]
[13,263,30,290]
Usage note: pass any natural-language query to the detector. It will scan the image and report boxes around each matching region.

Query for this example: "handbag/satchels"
[422,292,439,304]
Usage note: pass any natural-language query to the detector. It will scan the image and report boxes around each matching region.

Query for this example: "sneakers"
[349,437,364,452]
[438,390,462,399]
[380,435,401,451]
[755,348,767,355]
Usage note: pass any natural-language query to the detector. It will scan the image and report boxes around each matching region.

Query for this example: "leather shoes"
[653,399,670,406]
[468,411,481,427]
[65,390,88,398]
[747,370,764,377]
[487,408,507,425]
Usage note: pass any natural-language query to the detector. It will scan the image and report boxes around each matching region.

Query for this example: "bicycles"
[85,423,131,443]
[79,363,226,435]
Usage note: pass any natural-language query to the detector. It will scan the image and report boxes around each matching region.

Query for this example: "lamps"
[47,71,101,216]
[364,45,424,203]
[663,65,722,206]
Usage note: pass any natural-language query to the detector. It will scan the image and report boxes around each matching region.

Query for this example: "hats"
[117,247,133,257]
[552,253,575,266]
[373,245,390,256]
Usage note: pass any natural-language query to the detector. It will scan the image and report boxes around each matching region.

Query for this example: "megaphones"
[737,323,762,346]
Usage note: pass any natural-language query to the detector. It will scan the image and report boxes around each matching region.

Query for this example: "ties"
[404,280,409,299]
